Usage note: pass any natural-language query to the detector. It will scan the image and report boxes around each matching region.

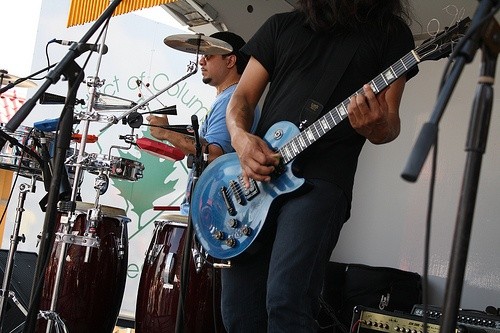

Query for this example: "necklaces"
[216,81,239,96]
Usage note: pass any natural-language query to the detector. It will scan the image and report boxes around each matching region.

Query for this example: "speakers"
[0,248,38,333]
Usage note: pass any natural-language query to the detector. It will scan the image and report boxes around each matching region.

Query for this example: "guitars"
[189,17,471,263]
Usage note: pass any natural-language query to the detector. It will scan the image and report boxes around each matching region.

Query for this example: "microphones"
[194,244,205,273]
[54,38,108,55]
[40,129,52,192]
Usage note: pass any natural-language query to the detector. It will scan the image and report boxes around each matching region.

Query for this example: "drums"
[35,201,129,333]
[88,152,144,181]
[0,123,58,177]
[58,147,89,187]
[135,215,221,333]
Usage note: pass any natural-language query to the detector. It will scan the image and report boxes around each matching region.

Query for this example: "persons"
[147,30,262,213]
[221,0,420,333]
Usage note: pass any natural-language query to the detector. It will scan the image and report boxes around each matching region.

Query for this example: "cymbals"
[163,34,233,57]
[0,72,38,89]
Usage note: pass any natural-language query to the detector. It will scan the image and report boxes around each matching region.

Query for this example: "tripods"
[9,16,108,333]
